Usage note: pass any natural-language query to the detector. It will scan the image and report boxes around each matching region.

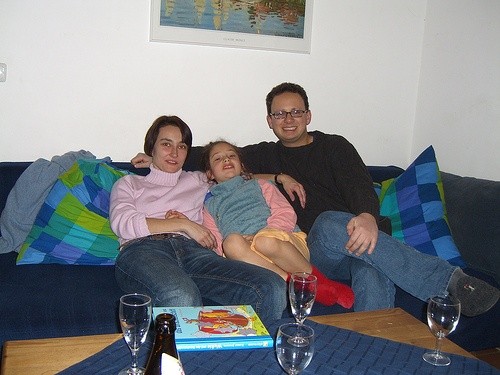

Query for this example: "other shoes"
[449,276,500,316]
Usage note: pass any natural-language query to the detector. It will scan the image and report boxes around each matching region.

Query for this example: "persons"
[132,82,500,317]
[110,116,287,324]
[164,140,353,309]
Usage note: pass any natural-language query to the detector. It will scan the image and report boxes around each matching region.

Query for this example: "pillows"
[373,145,471,271]
[15,160,134,266]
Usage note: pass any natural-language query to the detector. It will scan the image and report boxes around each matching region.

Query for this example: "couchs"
[0,162,500,353]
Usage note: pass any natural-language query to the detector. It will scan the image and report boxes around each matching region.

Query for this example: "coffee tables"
[0,308,489,375]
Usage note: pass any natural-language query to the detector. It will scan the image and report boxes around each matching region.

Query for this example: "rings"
[304,190,305,192]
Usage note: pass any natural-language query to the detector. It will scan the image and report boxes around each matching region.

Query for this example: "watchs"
[275,171,286,184]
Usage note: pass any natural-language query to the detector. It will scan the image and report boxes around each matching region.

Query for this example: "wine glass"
[423,295,461,366]
[119,293,152,375]
[287,272,317,347]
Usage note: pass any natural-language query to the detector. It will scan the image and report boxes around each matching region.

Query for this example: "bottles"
[142,314,186,374]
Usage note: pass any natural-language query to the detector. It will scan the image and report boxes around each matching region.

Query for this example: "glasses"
[270,110,308,119]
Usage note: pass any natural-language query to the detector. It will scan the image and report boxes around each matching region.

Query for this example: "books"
[147,305,274,351]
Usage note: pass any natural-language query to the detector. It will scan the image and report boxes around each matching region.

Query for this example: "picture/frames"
[149,0,315,54]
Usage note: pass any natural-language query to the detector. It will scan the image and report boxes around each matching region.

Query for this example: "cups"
[275,323,315,374]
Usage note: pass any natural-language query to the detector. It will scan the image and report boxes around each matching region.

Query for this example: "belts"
[152,233,180,240]
